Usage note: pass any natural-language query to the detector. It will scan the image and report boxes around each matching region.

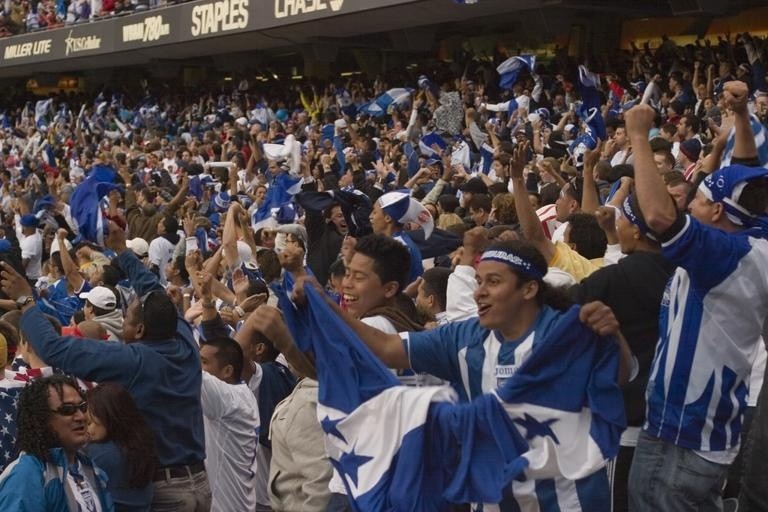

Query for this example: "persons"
[368,188,434,282]
[19,214,42,288]
[676,138,702,185]
[671,114,704,159]
[436,50,621,288]
[148,216,186,289]
[290,241,639,512]
[621,80,768,512]
[753,94,768,129]
[566,188,670,478]
[657,123,678,142]
[0,0,151,40]
[697,97,714,123]
[199,336,261,512]
[1,46,368,243]
[666,178,694,212]
[341,233,425,378]
[39,251,79,327]
[57,227,130,313]
[330,259,349,301]
[17,311,100,393]
[652,149,676,176]
[184,204,308,341]
[621,88,641,111]
[369,73,435,188]
[621,28,768,94]
[83,383,156,512]
[660,90,674,119]
[165,255,191,317]
[598,125,634,168]
[1,221,214,512]
[415,266,453,328]
[665,100,686,126]
[79,286,127,345]
[236,306,296,512]
[1,373,114,512]
[668,73,690,115]
[692,144,713,172]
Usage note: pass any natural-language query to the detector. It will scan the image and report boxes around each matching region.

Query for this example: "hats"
[378,187,434,241]
[423,158,440,166]
[679,138,702,162]
[79,286,117,311]
[459,178,488,195]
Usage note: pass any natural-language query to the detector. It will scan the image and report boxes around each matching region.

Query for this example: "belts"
[152,461,207,482]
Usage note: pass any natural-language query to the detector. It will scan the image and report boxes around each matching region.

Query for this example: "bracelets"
[235,304,246,320]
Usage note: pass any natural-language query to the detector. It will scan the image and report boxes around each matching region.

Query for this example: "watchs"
[13,294,34,312]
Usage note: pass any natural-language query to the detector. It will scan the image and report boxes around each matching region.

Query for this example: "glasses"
[139,290,152,324]
[50,402,88,417]
[568,176,578,193]
